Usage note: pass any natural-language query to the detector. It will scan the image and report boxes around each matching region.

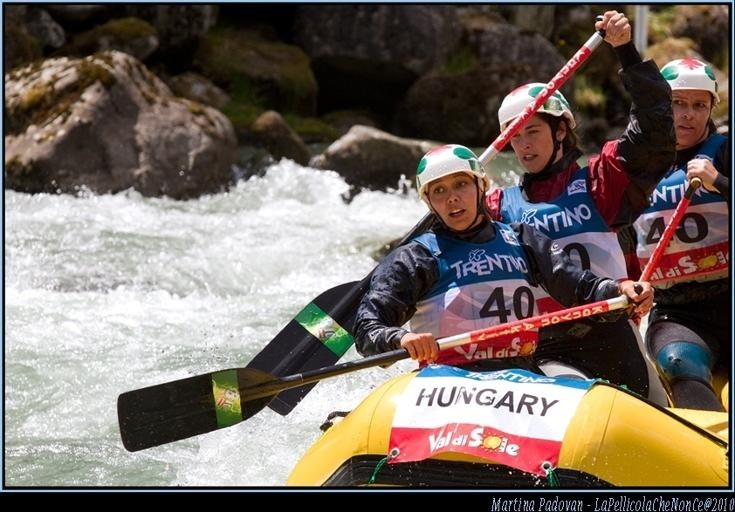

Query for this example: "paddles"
[245,15,607,416]
[118,284,642,453]
[578,177,701,386]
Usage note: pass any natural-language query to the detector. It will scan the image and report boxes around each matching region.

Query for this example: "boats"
[280,360,730,492]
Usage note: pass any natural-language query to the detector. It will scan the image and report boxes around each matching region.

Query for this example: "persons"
[638,58,729,412]
[352,144,655,395]
[483,9,669,407]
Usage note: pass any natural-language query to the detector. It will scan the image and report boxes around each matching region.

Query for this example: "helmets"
[660,58,721,103]
[498,83,577,134]
[416,144,490,204]
